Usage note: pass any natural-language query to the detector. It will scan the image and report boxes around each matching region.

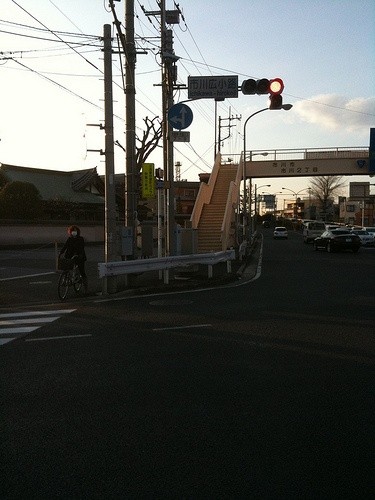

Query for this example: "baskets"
[58,260,74,270]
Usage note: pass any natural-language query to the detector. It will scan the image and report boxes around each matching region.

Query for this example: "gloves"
[72,256,77,260]
[58,253,62,257]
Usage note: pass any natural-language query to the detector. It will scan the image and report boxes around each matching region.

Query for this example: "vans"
[302,222,326,244]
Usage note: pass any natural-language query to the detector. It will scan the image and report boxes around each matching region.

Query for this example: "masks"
[72,231,77,236]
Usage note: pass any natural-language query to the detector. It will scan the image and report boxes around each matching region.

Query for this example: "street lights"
[241,104,293,243]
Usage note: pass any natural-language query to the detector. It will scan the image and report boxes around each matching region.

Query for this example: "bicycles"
[57,254,84,300]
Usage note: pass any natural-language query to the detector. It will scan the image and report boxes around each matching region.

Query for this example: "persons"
[58,226,88,295]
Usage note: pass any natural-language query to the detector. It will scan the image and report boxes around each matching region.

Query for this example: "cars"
[262,220,270,224]
[272,226,288,239]
[313,229,362,253]
[302,219,375,248]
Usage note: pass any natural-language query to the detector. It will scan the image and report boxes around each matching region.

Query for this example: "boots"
[83,280,88,289]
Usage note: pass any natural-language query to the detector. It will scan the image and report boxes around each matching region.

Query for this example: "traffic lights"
[241,78,283,96]
